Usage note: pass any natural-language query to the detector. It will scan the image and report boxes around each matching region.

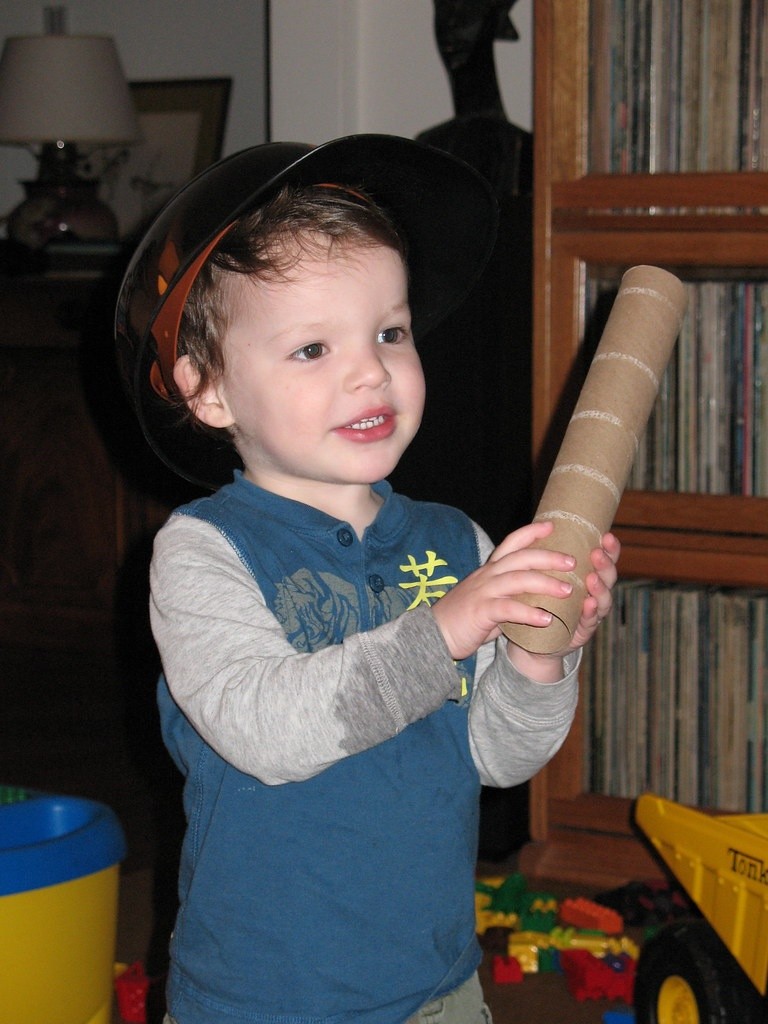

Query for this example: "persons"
[113,133,621,1024]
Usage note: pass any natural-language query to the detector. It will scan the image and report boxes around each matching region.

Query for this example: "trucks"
[633,794,767,1023]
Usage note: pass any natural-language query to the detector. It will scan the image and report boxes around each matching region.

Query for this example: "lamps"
[0,34,138,252]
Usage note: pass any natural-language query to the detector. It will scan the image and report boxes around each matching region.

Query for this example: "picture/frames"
[112,76,231,233]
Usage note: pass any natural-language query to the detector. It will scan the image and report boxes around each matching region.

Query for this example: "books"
[587,0,768,173]
[584,576,768,817]
[589,277,768,498]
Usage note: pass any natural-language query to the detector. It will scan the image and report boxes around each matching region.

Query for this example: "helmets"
[115,132,501,488]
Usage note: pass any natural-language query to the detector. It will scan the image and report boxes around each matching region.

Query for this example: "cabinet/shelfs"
[511,0,768,890]
[0,255,166,657]
